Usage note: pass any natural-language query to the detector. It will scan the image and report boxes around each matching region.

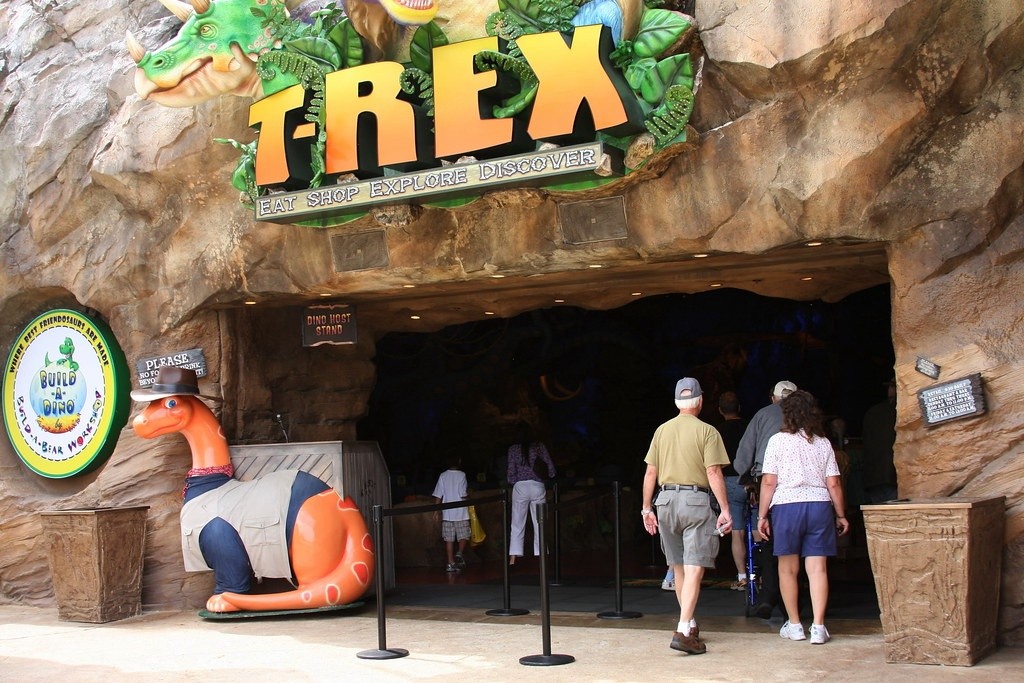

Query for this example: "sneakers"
[662,578,675,591]
[779,621,807,641]
[808,624,830,643]
[689,624,701,639]
[730,578,749,591]
[669,631,706,654]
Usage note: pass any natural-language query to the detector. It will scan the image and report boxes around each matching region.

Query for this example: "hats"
[773,380,798,398]
[675,377,704,400]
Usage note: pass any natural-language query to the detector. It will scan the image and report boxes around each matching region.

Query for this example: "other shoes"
[455,552,465,569]
[757,606,770,619]
[446,562,460,571]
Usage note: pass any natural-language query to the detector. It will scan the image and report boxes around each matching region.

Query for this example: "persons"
[432,454,471,572]
[641,377,733,655]
[757,390,849,643]
[507,421,556,568]
[716,380,865,591]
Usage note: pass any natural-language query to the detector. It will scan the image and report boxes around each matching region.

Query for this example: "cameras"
[713,524,726,537]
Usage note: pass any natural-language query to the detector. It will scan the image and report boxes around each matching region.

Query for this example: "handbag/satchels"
[533,456,559,491]
[468,505,486,547]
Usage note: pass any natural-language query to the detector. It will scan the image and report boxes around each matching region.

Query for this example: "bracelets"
[836,515,846,518]
[757,517,768,519]
[641,508,653,515]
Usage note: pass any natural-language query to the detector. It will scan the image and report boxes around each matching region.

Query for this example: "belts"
[660,484,711,495]
